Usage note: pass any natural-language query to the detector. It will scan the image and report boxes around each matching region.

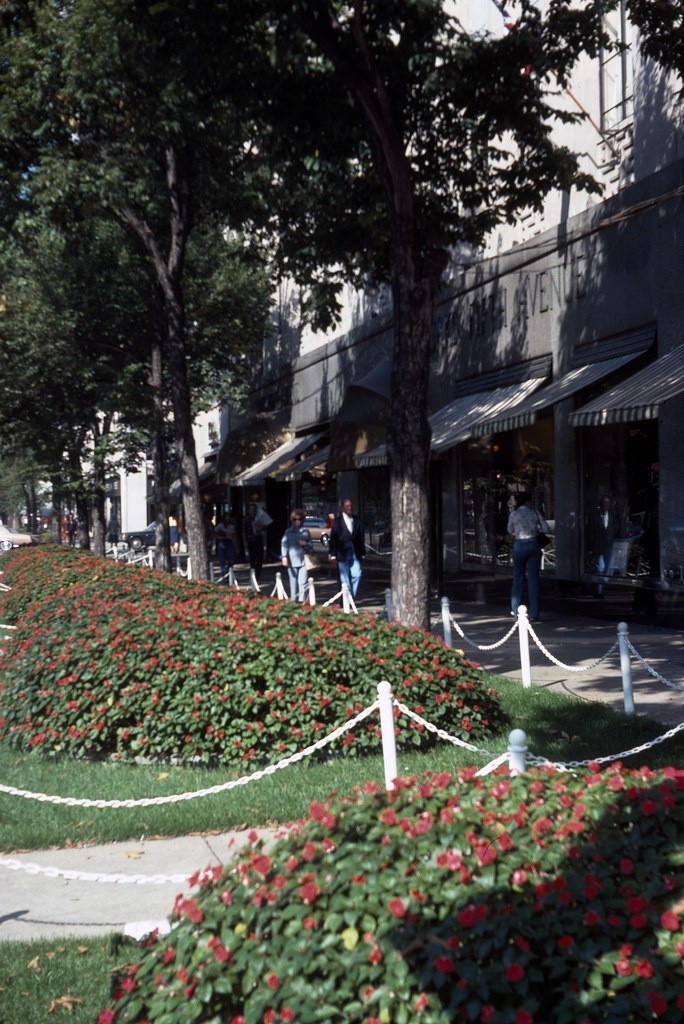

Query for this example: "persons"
[281,509,313,604]
[215,512,239,577]
[587,497,620,594]
[506,490,550,622]
[152,516,188,572]
[244,503,264,580]
[66,514,121,548]
[328,498,366,600]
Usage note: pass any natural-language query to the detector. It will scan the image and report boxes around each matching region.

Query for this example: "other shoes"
[530,617,536,622]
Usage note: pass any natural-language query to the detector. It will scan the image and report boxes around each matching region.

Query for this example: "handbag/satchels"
[255,510,273,527]
[303,544,322,572]
[534,511,551,548]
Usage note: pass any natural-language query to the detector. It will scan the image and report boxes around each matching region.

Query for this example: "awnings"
[276,445,330,482]
[148,460,219,505]
[467,347,649,439]
[566,342,684,427]
[352,375,548,468]
[228,430,326,486]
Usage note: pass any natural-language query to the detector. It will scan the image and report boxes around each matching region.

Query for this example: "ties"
[603,511,609,529]
[348,517,352,533]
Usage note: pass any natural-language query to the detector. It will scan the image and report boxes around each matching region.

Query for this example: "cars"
[303,515,332,546]
[0,525,40,552]
[121,521,178,551]
